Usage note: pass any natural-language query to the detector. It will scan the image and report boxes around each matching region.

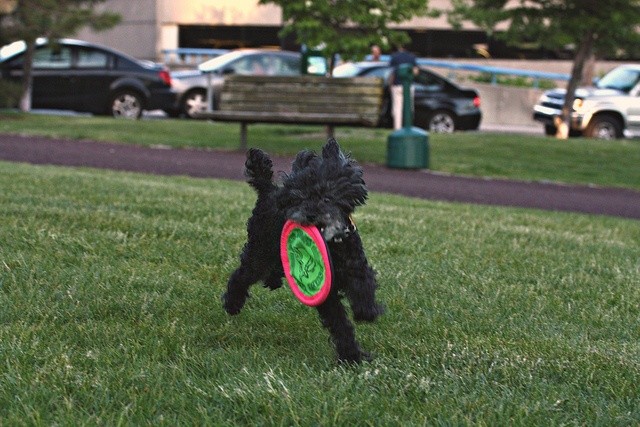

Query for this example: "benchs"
[191,73,393,154]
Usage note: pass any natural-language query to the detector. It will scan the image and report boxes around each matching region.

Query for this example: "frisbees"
[280,218,332,307]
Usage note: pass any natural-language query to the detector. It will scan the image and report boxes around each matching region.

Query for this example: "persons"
[371,45,381,61]
[389,43,420,131]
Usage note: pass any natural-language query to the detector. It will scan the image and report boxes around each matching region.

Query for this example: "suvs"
[533,64,640,138]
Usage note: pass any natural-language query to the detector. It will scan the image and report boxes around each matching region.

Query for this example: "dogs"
[222,136,386,365]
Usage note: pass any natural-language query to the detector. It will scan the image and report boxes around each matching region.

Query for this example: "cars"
[330,60,482,136]
[172,48,327,118]
[0,37,171,122]
